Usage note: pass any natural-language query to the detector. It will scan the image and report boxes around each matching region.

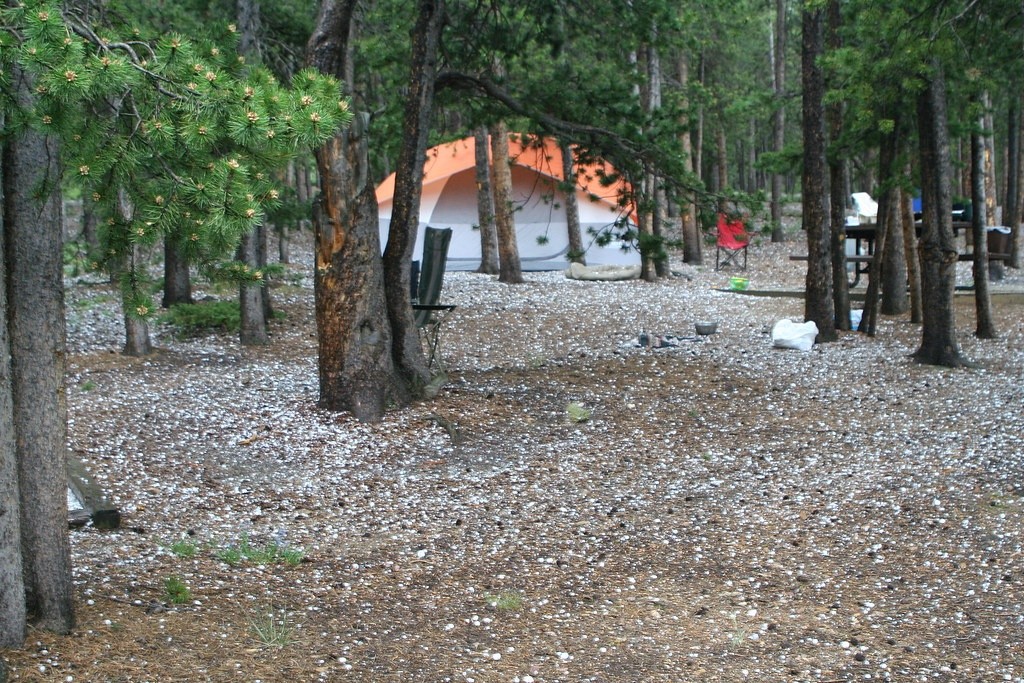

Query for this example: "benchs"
[953,250,1013,301]
[788,250,875,294]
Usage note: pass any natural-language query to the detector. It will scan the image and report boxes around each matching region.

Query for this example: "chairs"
[714,208,753,274]
[412,226,459,377]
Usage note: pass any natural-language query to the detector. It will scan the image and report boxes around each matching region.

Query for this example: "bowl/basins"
[694,321,718,335]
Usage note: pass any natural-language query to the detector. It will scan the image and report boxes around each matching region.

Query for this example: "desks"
[843,217,975,293]
